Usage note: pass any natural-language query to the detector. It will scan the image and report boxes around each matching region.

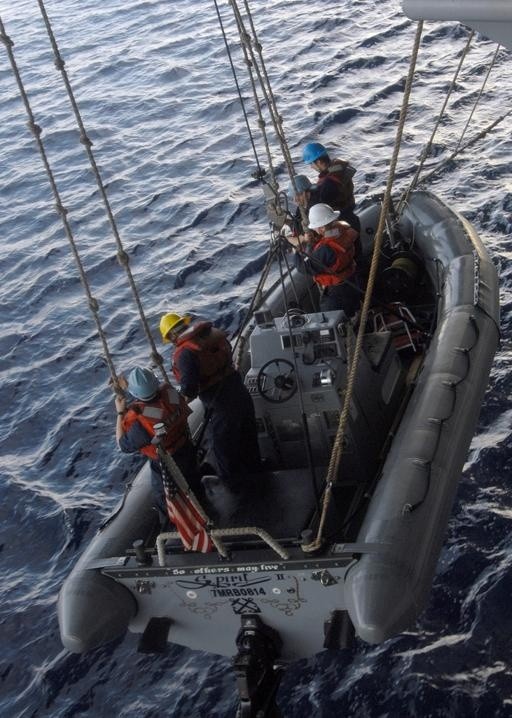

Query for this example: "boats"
[54,188,499,711]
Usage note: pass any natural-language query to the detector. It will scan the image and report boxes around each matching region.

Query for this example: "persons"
[293,205,362,317]
[279,176,329,238]
[109,366,217,540]
[304,142,363,270]
[158,307,261,475]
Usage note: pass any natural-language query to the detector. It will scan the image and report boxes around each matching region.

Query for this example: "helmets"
[288,176,313,196]
[124,368,159,402]
[308,202,341,230]
[304,144,326,164]
[160,313,192,344]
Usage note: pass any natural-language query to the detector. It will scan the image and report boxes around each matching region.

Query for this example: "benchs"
[191,466,332,538]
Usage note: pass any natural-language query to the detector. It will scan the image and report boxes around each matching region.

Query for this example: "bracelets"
[115,412,126,416]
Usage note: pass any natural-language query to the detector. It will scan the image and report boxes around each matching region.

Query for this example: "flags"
[149,444,214,554]
